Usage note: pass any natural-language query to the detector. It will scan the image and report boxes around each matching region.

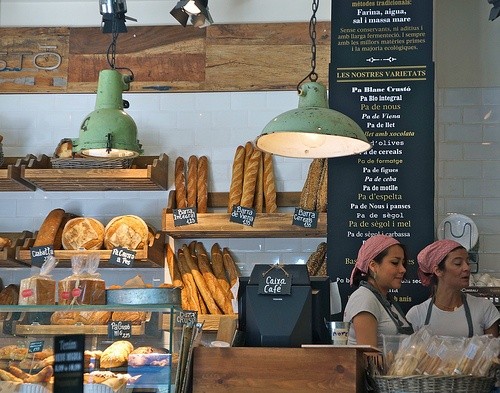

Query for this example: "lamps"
[254,0,373,160]
[169,0,214,28]
[72,0,144,159]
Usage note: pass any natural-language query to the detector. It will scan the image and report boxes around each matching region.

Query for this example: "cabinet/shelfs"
[160,190,329,334]
[0,153,169,339]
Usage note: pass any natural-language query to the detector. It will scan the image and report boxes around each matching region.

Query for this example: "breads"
[164,239,239,315]
[0,208,172,325]
[0,344,54,383]
[307,242,328,276]
[83,341,170,391]
[175,142,328,214]
[56,142,73,157]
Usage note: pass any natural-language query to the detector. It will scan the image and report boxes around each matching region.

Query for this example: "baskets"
[367,371,497,393]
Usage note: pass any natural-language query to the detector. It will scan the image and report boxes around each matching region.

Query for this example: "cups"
[330,322,351,345]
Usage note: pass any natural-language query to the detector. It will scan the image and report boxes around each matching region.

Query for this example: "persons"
[404,240,500,339]
[343,235,414,355]
